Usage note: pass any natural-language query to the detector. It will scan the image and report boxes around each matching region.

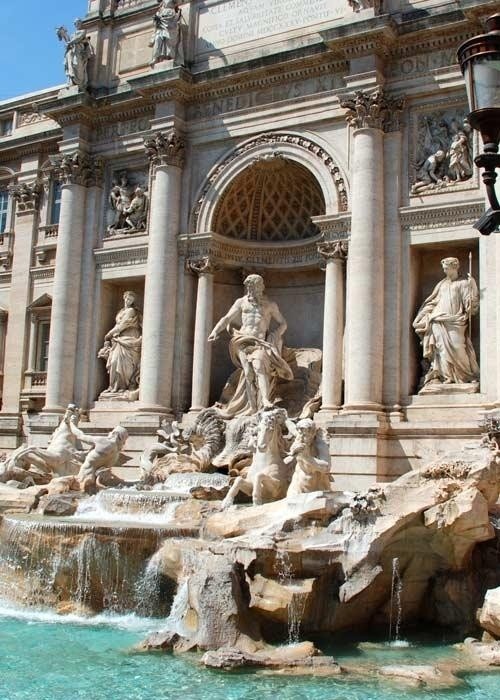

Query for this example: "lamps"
[457,12,500,235]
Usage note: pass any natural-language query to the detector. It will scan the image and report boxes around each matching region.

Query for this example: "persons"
[412,257,481,384]
[103,289,143,391]
[63,17,93,85]
[207,273,288,415]
[150,0,184,68]
[411,116,473,194]
[111,177,150,233]
